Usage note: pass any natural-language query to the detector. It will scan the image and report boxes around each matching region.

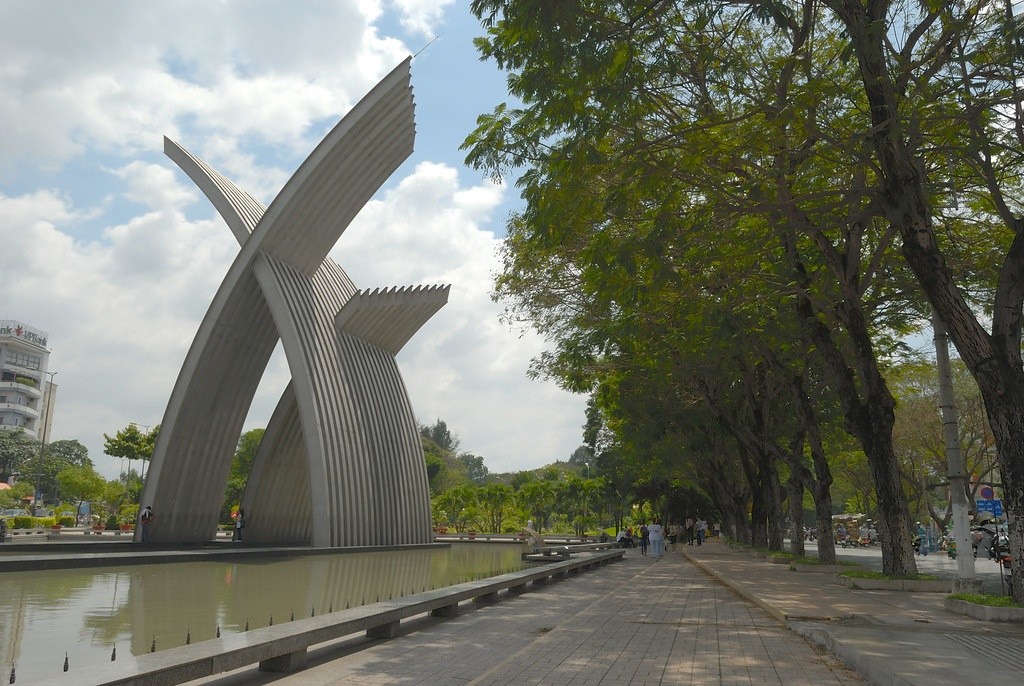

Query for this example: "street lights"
[25,366,58,515]
[130,422,148,477]
[585,462,589,479]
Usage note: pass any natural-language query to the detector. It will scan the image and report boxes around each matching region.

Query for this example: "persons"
[236,509,246,543]
[803,521,950,558]
[685,515,721,546]
[600,517,684,562]
[140,506,156,544]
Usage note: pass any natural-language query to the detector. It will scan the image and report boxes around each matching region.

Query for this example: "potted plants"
[458,504,481,539]
[512,509,538,540]
[120,521,133,530]
[91,498,107,535]
[573,515,598,542]
[43,502,78,534]
[437,510,450,534]
